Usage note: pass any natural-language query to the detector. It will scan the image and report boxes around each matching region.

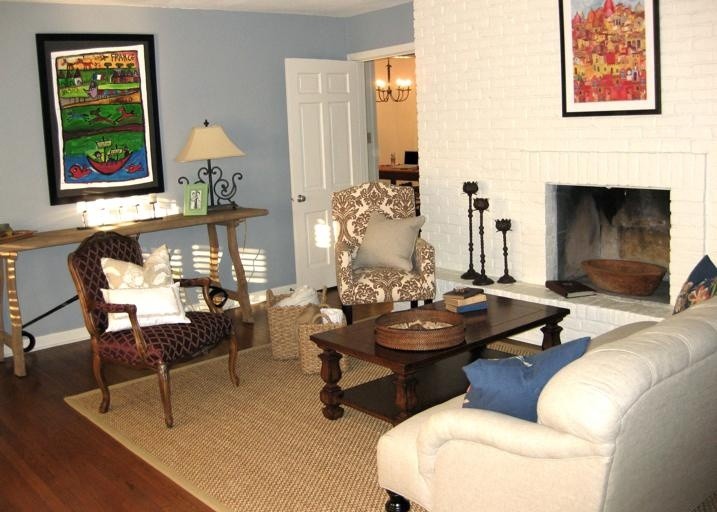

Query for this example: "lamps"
[176,121,243,209]
[374,57,412,102]
[461,181,517,287]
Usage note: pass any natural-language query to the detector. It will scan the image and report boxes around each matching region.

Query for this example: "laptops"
[396,150,418,168]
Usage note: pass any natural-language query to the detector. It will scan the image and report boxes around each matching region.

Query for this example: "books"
[444,301,487,313]
[544,280,596,298]
[442,294,486,307]
[442,286,484,299]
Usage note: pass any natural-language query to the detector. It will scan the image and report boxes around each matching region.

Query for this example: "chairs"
[329,182,436,324]
[404,150,418,164]
[67,231,238,426]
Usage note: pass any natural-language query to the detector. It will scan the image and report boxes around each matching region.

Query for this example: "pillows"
[462,335,591,423]
[671,254,717,315]
[353,211,425,272]
[96,281,191,332]
[101,243,174,288]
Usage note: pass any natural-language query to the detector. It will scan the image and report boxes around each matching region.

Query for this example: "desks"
[0,204,268,378]
[378,164,419,184]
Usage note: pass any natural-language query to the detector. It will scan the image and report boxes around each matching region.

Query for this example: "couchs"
[374,292,717,512]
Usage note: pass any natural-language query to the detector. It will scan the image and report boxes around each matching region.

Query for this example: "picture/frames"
[35,32,163,204]
[182,183,208,216]
[557,0,662,115]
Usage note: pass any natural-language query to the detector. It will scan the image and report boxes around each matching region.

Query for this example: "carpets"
[67,311,570,512]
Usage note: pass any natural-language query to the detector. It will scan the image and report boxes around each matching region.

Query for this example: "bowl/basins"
[580,260,666,296]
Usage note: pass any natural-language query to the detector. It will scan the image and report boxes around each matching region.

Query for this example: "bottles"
[391,152,396,167]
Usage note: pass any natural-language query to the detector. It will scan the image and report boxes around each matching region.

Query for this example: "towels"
[273,285,319,308]
[319,306,343,325]
